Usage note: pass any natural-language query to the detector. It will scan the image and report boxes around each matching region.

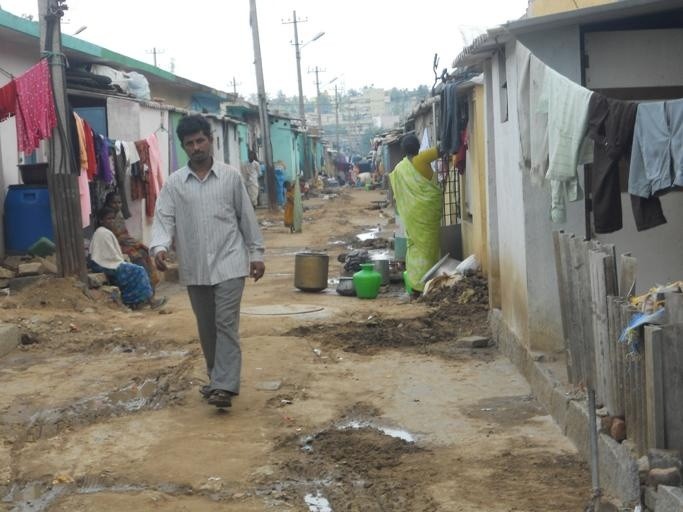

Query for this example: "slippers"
[150,295,171,310]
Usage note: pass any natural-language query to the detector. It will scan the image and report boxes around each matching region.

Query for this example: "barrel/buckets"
[366,259,388,285]
[293,253,329,292]
[3,184,54,255]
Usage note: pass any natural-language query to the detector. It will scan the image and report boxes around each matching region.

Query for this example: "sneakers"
[199,383,232,408]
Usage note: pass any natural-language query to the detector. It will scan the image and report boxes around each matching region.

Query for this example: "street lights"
[298,32,324,50]
[65,23,91,46]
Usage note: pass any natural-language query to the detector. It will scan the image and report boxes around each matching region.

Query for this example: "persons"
[387,131,449,302]
[104,190,159,295]
[85,206,166,310]
[239,149,261,211]
[281,173,301,233]
[147,113,267,407]
